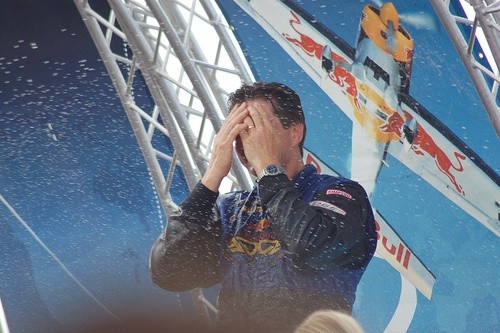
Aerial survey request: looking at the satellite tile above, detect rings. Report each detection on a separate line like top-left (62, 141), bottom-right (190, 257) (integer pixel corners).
top-left (246, 125), bottom-right (255, 131)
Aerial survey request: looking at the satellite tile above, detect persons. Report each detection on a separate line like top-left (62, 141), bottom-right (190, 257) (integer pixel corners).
top-left (149, 83), bottom-right (378, 333)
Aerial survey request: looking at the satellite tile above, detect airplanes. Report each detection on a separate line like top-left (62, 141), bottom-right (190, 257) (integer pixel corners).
top-left (232, 0), bottom-right (500, 302)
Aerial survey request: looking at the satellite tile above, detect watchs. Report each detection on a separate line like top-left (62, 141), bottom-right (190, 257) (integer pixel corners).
top-left (256, 163), bottom-right (288, 183)
